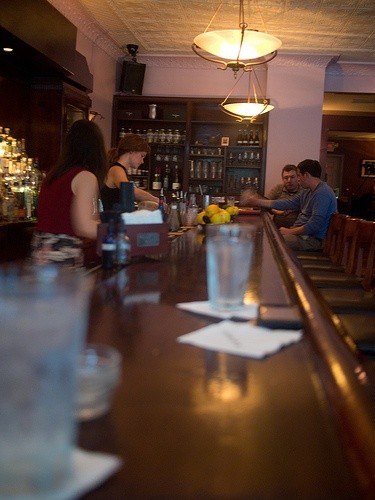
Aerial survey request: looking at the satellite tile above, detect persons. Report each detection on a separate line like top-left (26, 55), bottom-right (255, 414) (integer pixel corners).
top-left (240, 159), bottom-right (337, 250)
top-left (34, 119), bottom-right (106, 241)
top-left (264, 164), bottom-right (307, 229)
top-left (95, 134), bottom-right (165, 222)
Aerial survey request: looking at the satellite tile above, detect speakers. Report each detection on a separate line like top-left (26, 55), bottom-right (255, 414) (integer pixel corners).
top-left (119, 61), bottom-right (146, 95)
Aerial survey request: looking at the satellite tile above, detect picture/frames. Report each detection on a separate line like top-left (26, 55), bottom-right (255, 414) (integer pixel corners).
top-left (362, 160), bottom-right (375, 177)
top-left (221, 137), bottom-right (229, 146)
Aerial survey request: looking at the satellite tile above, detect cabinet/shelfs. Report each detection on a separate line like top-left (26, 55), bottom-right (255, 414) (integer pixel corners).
top-left (111, 95), bottom-right (270, 198)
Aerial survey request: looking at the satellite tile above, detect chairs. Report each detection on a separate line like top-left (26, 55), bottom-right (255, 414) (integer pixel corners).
top-left (291, 211), bottom-right (375, 352)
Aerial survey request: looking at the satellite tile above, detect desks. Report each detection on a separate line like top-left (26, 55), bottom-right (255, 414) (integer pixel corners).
top-left (0, 206), bottom-right (375, 500)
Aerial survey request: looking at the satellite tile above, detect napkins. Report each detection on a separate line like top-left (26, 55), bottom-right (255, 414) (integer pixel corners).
top-left (175, 319), bottom-right (302, 358)
top-left (175, 296), bottom-right (261, 323)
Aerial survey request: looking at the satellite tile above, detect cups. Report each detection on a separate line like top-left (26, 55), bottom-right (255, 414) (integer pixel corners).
top-left (0, 266), bottom-right (95, 497)
top-left (189, 193), bottom-right (196, 206)
top-left (204, 223), bottom-right (258, 312)
top-left (202, 195), bottom-right (209, 209)
top-left (226, 196), bottom-right (235, 207)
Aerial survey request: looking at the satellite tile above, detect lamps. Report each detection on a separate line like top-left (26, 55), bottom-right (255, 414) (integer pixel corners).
top-left (218, 65), bottom-right (276, 124)
top-left (192, 0), bottom-right (283, 77)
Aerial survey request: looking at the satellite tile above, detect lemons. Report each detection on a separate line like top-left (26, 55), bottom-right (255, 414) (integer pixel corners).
top-left (196, 204), bottom-right (238, 225)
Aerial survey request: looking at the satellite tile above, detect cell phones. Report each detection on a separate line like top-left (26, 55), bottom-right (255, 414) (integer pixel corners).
top-left (258, 303), bottom-right (303, 330)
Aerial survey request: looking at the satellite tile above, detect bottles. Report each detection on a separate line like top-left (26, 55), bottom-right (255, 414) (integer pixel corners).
top-left (95, 211), bottom-right (131, 269)
top-left (149, 104), bottom-right (157, 120)
top-left (0, 127), bottom-right (46, 221)
top-left (119, 127), bottom-right (261, 233)
